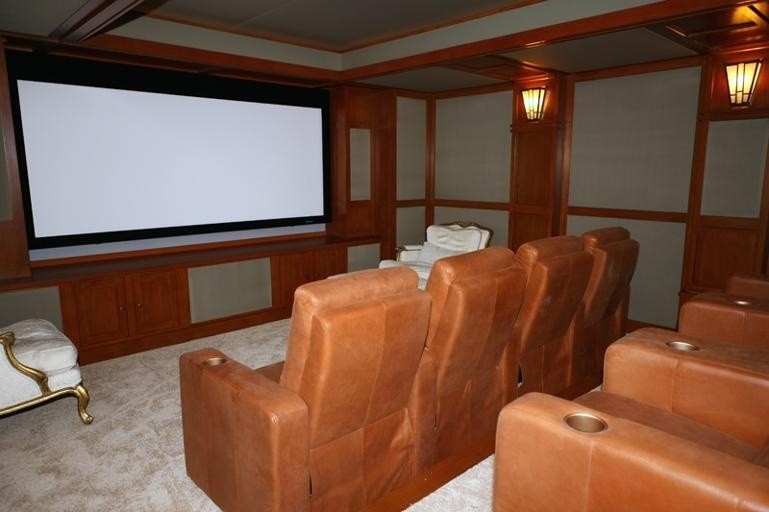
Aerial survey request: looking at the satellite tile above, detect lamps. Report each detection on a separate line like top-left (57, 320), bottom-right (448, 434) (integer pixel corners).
top-left (723, 59), bottom-right (765, 109)
top-left (521, 85), bottom-right (549, 123)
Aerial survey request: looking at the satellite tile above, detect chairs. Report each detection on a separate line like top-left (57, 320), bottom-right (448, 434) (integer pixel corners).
top-left (0, 316), bottom-right (94, 424)
top-left (411, 245), bottom-right (530, 506)
top-left (569, 225), bottom-right (641, 401)
top-left (377, 223), bottom-right (492, 291)
top-left (491, 335), bottom-right (768, 511)
top-left (690, 271), bottom-right (768, 311)
top-left (179, 267), bottom-right (433, 511)
top-left (516, 235), bottom-right (595, 400)
top-left (642, 297), bottom-right (768, 370)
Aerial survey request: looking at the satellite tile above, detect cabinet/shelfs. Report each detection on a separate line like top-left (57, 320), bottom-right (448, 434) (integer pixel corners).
top-left (59, 263), bottom-right (185, 353)
